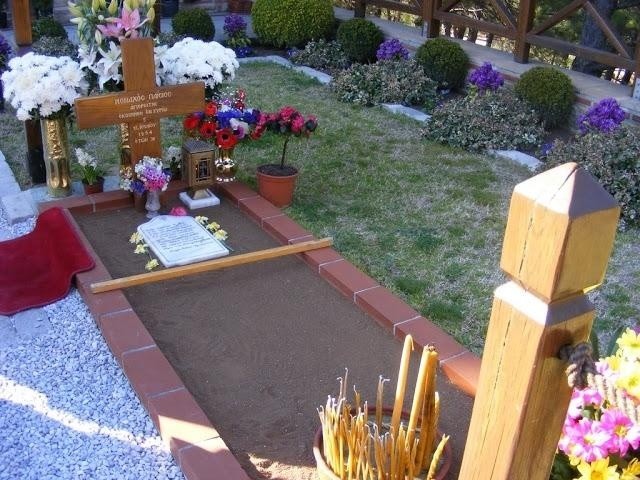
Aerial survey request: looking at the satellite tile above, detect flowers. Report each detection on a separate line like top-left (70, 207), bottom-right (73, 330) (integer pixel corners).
top-left (129, 231), bottom-right (159, 273)
top-left (169, 207), bottom-right (187, 216)
top-left (0, 0), bottom-right (318, 196)
top-left (195, 215), bottom-right (234, 253)
top-left (558, 327), bottom-right (640, 480)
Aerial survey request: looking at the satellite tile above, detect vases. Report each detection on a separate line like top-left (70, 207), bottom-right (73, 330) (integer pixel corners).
top-left (313, 406), bottom-right (453, 480)
top-left (257, 164), bottom-right (299, 210)
top-left (145, 192), bottom-right (161, 218)
top-left (134, 193), bottom-right (145, 211)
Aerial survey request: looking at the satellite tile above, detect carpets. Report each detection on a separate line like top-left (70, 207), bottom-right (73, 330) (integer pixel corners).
top-left (0, 207), bottom-right (95, 315)
